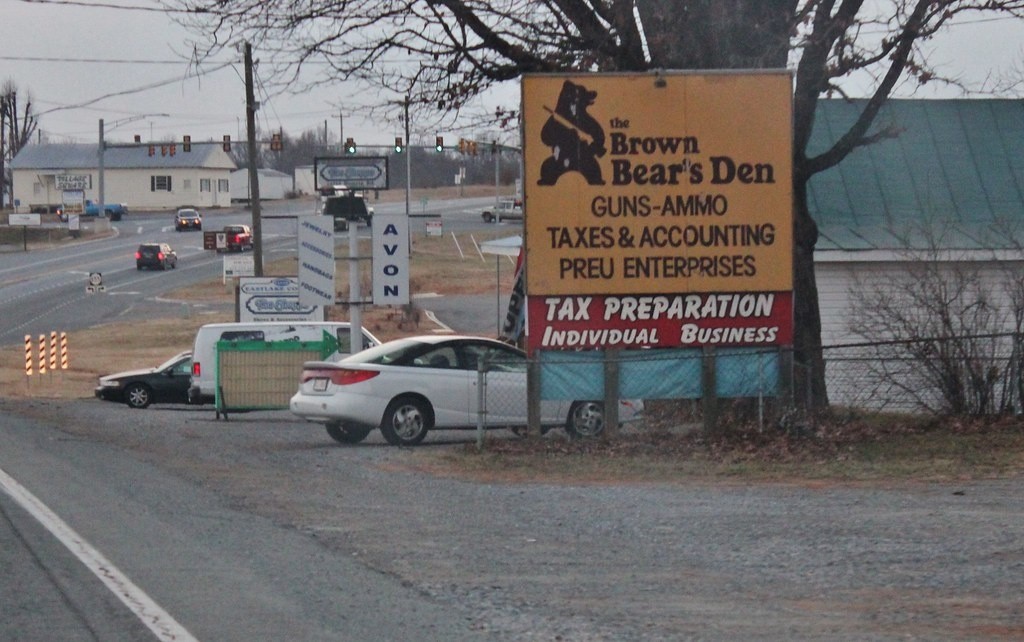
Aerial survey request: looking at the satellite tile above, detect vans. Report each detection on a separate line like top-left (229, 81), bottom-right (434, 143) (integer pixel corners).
top-left (188, 320), bottom-right (424, 405)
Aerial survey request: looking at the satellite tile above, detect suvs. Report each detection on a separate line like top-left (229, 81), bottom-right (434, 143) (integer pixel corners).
top-left (137, 243), bottom-right (177, 271)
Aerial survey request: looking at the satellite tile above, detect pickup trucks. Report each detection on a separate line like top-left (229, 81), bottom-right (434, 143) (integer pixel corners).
top-left (56, 198), bottom-right (128, 221)
top-left (217, 224), bottom-right (253, 254)
top-left (479, 200), bottom-right (522, 223)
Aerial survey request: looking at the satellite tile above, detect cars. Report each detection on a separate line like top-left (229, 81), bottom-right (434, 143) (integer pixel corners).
top-left (95, 350), bottom-right (194, 410)
top-left (174, 209), bottom-right (202, 232)
top-left (288, 334), bottom-right (646, 447)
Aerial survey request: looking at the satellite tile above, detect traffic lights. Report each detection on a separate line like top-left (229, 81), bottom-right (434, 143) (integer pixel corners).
top-left (345, 138), bottom-right (356, 153)
top-left (436, 136), bottom-right (443, 152)
top-left (395, 137), bottom-right (402, 153)
